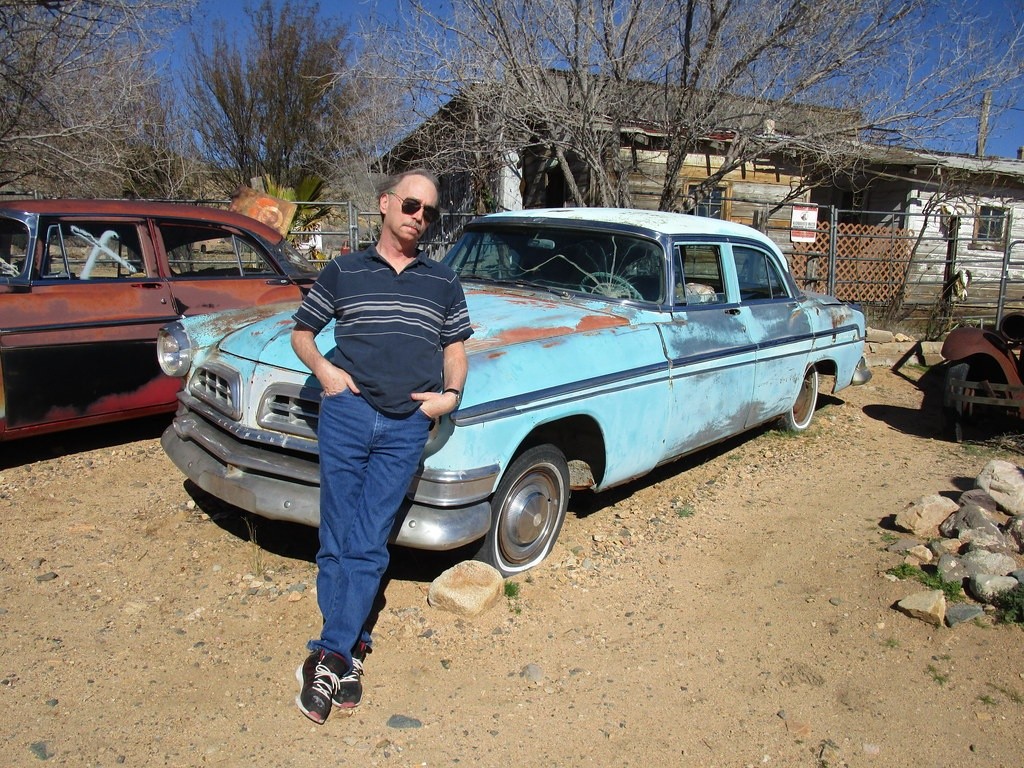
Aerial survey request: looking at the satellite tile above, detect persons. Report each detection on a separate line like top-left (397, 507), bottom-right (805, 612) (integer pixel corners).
top-left (290, 168), bottom-right (476, 725)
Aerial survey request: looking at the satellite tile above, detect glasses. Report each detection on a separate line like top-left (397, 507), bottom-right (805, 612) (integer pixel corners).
top-left (389, 191), bottom-right (441, 222)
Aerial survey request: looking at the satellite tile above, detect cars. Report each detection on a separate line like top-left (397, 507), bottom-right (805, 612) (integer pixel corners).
top-left (0, 191), bottom-right (318, 443)
top-left (157, 207), bottom-right (873, 579)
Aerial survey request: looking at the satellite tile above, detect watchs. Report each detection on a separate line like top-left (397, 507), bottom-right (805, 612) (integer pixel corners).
top-left (441, 388), bottom-right (461, 407)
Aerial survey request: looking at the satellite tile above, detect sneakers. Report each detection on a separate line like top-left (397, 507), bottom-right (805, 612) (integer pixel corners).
top-left (332, 639), bottom-right (372, 708)
top-left (294, 648), bottom-right (350, 725)
